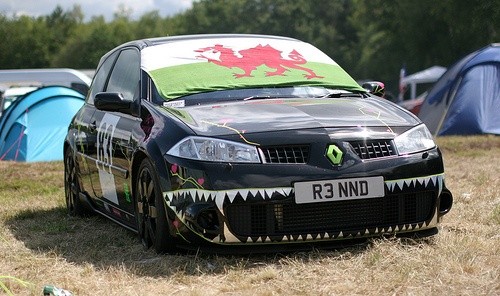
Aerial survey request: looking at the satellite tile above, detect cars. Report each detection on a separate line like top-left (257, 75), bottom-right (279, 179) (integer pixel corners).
top-left (61, 32), bottom-right (453, 256)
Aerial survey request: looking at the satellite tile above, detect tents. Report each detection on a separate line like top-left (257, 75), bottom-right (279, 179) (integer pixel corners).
top-left (0, 85), bottom-right (88, 163)
top-left (401, 65), bottom-right (449, 100)
top-left (418, 42), bottom-right (500, 137)
